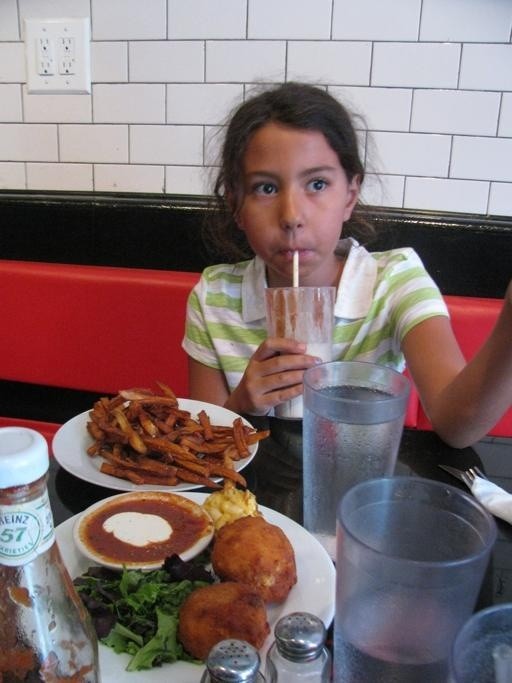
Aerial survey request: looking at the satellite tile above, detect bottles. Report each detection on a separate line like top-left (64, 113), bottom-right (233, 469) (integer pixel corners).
top-left (0, 426), bottom-right (98, 683)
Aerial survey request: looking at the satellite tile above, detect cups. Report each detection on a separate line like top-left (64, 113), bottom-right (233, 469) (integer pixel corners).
top-left (264, 286), bottom-right (335, 425)
top-left (449, 605), bottom-right (511, 683)
top-left (294, 361), bottom-right (412, 566)
top-left (331, 476), bottom-right (499, 681)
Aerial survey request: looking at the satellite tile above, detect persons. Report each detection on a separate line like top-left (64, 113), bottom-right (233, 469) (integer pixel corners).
top-left (179, 76), bottom-right (512, 453)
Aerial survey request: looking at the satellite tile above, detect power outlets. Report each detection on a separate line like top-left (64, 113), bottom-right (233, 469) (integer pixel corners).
top-left (23, 17), bottom-right (92, 96)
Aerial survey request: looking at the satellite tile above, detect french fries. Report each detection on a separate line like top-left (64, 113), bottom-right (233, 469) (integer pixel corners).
top-left (87, 376), bottom-right (272, 490)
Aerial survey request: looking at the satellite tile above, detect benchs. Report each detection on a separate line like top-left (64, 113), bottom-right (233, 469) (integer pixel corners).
top-left (1, 188), bottom-right (512, 452)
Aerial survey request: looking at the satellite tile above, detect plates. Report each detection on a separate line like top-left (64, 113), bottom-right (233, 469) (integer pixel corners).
top-left (51, 398), bottom-right (261, 492)
top-left (52, 488), bottom-right (335, 680)
